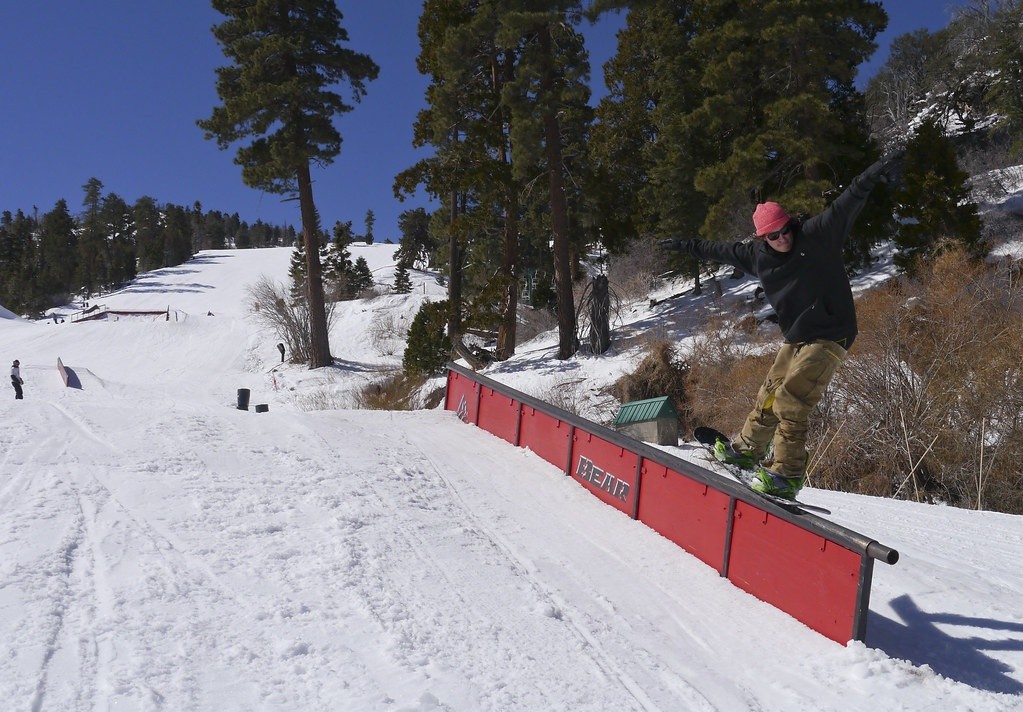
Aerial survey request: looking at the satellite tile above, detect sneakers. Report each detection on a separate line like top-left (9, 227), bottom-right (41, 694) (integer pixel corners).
top-left (714, 438), bottom-right (769, 470)
top-left (751, 451), bottom-right (809, 501)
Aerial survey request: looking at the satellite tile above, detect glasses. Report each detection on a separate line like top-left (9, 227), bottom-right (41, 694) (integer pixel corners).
top-left (764, 223), bottom-right (789, 241)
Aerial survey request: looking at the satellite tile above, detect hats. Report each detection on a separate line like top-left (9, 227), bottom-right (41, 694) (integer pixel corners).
top-left (752, 201), bottom-right (790, 236)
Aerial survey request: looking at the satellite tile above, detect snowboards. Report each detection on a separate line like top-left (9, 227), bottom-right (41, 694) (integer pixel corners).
top-left (692, 425), bottom-right (834, 516)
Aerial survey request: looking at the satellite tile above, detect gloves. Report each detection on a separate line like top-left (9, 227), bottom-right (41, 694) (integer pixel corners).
top-left (857, 150), bottom-right (903, 191)
top-left (655, 238), bottom-right (688, 253)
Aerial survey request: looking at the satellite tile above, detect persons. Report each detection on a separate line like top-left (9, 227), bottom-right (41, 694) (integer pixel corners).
top-left (61, 318), bottom-right (65, 323)
top-left (53, 313), bottom-right (58, 324)
top-left (10, 360), bottom-right (23, 399)
top-left (656, 150), bottom-right (905, 499)
